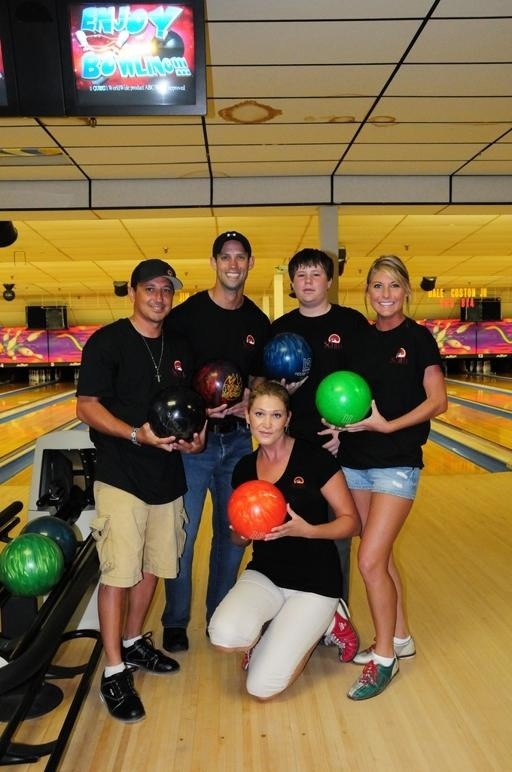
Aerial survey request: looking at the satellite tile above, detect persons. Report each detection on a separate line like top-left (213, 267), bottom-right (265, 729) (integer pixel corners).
top-left (336, 254), bottom-right (449, 700)
top-left (161, 229), bottom-right (274, 654)
top-left (272, 249), bottom-right (372, 643)
top-left (74, 257), bottom-right (210, 725)
top-left (207, 380), bottom-right (360, 701)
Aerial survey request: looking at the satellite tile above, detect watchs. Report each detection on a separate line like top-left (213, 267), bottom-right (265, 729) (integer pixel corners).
top-left (130, 428), bottom-right (142, 447)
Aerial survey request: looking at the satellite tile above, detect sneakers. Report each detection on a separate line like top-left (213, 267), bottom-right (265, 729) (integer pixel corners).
top-left (348, 658), bottom-right (401, 699)
top-left (355, 638), bottom-right (418, 663)
top-left (98, 668), bottom-right (146, 723)
top-left (123, 637), bottom-right (181, 675)
top-left (325, 600), bottom-right (357, 663)
top-left (163, 627), bottom-right (191, 655)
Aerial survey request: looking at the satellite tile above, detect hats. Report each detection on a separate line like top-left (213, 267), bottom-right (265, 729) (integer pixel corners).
top-left (214, 232), bottom-right (252, 254)
top-left (132, 260), bottom-right (185, 291)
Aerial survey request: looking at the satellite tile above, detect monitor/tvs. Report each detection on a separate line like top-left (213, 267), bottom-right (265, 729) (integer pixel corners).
top-left (57, 1), bottom-right (207, 117)
top-left (1, 7), bottom-right (20, 120)
top-left (26, 306), bottom-right (67, 331)
top-left (461, 299), bottom-right (502, 322)
